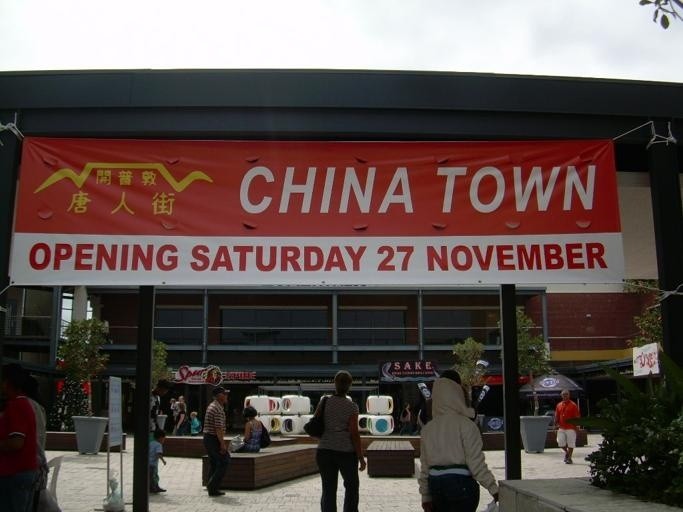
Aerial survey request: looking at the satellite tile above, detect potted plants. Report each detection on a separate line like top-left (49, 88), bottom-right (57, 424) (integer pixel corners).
top-left (149, 337), bottom-right (168, 432)
top-left (515, 306), bottom-right (558, 454)
top-left (56, 317), bottom-right (112, 455)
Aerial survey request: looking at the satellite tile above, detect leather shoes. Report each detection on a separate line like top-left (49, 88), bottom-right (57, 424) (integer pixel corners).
top-left (207, 482), bottom-right (225, 496)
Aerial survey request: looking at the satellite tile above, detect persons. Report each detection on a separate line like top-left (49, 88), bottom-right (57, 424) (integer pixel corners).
top-left (174, 394), bottom-right (186, 419)
top-left (553, 390), bottom-right (579, 464)
top-left (313, 370), bottom-right (367, 511)
top-left (0, 362), bottom-right (39, 512)
top-left (399, 403), bottom-right (413, 436)
top-left (23, 375), bottom-right (49, 512)
top-left (203, 387), bottom-right (231, 495)
top-left (147, 429), bottom-right (167, 492)
top-left (419, 371), bottom-right (476, 425)
top-left (413, 404), bottom-right (424, 436)
top-left (419, 379), bottom-right (499, 510)
top-left (147, 379), bottom-right (170, 492)
top-left (230, 407), bottom-right (262, 452)
top-left (172, 411), bottom-right (190, 435)
top-left (188, 411), bottom-right (201, 435)
top-left (169, 397), bottom-right (178, 416)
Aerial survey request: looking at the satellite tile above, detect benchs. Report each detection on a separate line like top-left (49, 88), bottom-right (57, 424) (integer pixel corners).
top-left (163, 433), bottom-right (321, 491)
top-left (480, 429), bottom-right (586, 451)
top-left (360, 434), bottom-right (421, 479)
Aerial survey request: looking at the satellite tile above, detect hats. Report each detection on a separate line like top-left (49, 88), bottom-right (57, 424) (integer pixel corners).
top-left (212, 387), bottom-right (230, 396)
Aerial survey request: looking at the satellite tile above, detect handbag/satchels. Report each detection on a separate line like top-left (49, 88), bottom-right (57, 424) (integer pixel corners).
top-left (195, 425), bottom-right (203, 432)
top-left (303, 396), bottom-right (327, 438)
top-left (260, 422), bottom-right (272, 448)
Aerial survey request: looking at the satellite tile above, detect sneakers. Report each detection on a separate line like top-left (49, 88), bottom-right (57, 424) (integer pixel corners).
top-left (564, 453), bottom-right (572, 464)
top-left (150, 484), bottom-right (167, 492)
